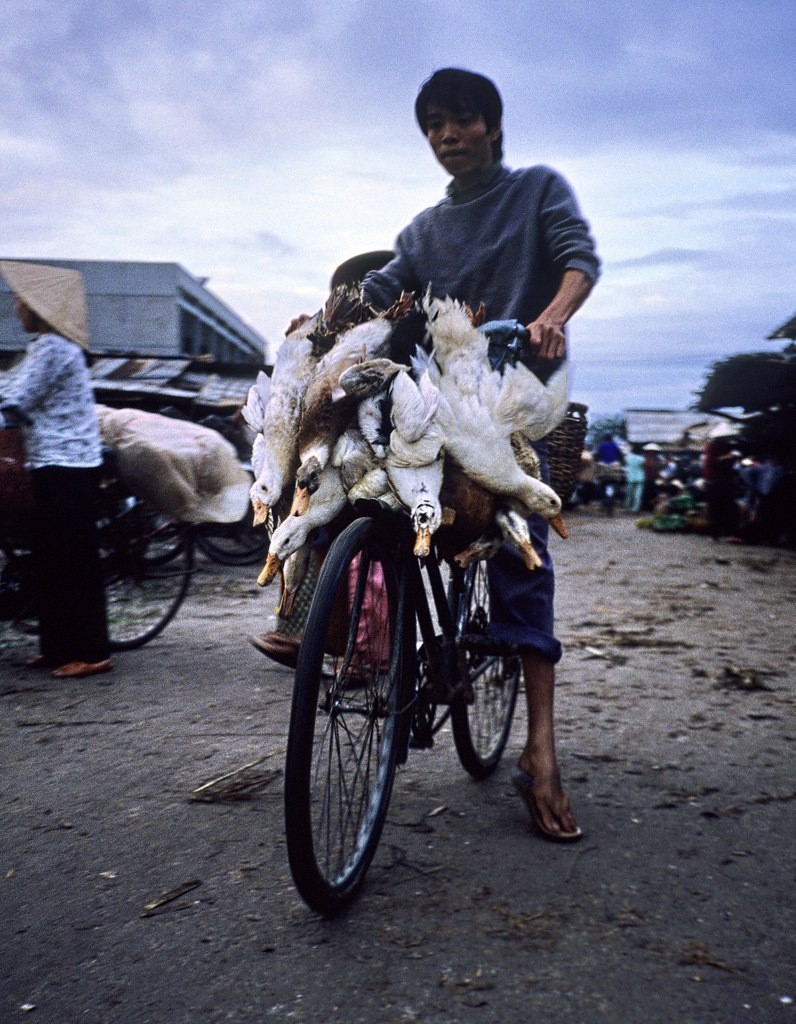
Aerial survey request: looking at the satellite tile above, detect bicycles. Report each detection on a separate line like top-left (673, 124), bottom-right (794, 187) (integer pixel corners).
top-left (280, 316), bottom-right (537, 920)
top-left (1, 443), bottom-right (271, 652)
top-left (592, 461), bottom-right (629, 516)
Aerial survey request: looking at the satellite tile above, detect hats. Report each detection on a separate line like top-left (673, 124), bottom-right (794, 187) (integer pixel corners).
top-left (643, 443), bottom-right (662, 452)
top-left (709, 423), bottom-right (740, 438)
top-left (0, 260), bottom-right (89, 352)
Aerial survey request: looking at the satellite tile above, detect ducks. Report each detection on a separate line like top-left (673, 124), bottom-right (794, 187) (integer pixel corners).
top-left (241, 280), bottom-right (573, 619)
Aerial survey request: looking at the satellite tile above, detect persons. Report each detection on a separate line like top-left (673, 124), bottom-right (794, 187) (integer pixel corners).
top-left (643, 443), bottom-right (662, 511)
top-left (702, 423), bottom-right (740, 544)
top-left (246, 69), bottom-right (603, 840)
top-left (0, 262), bottom-right (116, 677)
top-left (624, 447), bottom-right (645, 512)
top-left (594, 430), bottom-right (622, 462)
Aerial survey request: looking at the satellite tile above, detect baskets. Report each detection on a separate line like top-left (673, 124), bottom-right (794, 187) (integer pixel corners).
top-left (541, 403), bottom-right (588, 505)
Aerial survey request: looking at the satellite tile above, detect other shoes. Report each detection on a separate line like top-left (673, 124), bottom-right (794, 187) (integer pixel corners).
top-left (51, 657), bottom-right (112, 676)
top-left (25, 654), bottom-right (69, 665)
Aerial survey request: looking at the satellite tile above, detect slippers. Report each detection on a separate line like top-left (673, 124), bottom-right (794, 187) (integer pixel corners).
top-left (246, 632), bottom-right (302, 668)
top-left (512, 765), bottom-right (583, 841)
